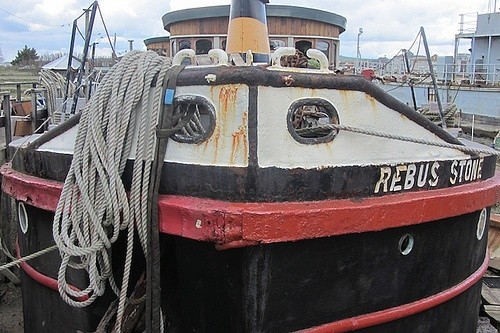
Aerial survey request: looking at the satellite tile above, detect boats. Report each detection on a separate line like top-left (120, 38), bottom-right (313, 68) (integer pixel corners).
top-left (0, 0), bottom-right (499, 333)
